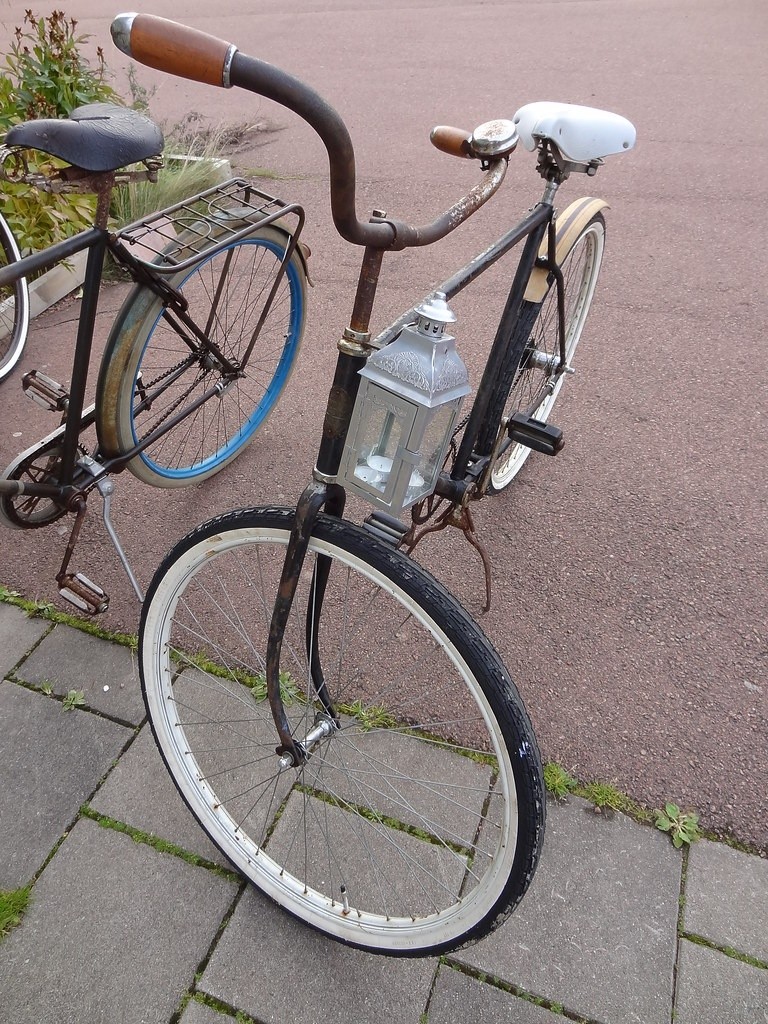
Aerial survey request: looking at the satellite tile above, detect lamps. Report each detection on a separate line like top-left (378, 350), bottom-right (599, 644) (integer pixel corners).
top-left (331, 290), bottom-right (474, 519)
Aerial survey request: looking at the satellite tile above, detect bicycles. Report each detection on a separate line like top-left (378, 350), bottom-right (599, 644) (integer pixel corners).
top-left (108, 10), bottom-right (638, 958)
top-left (0, 102), bottom-right (316, 616)
